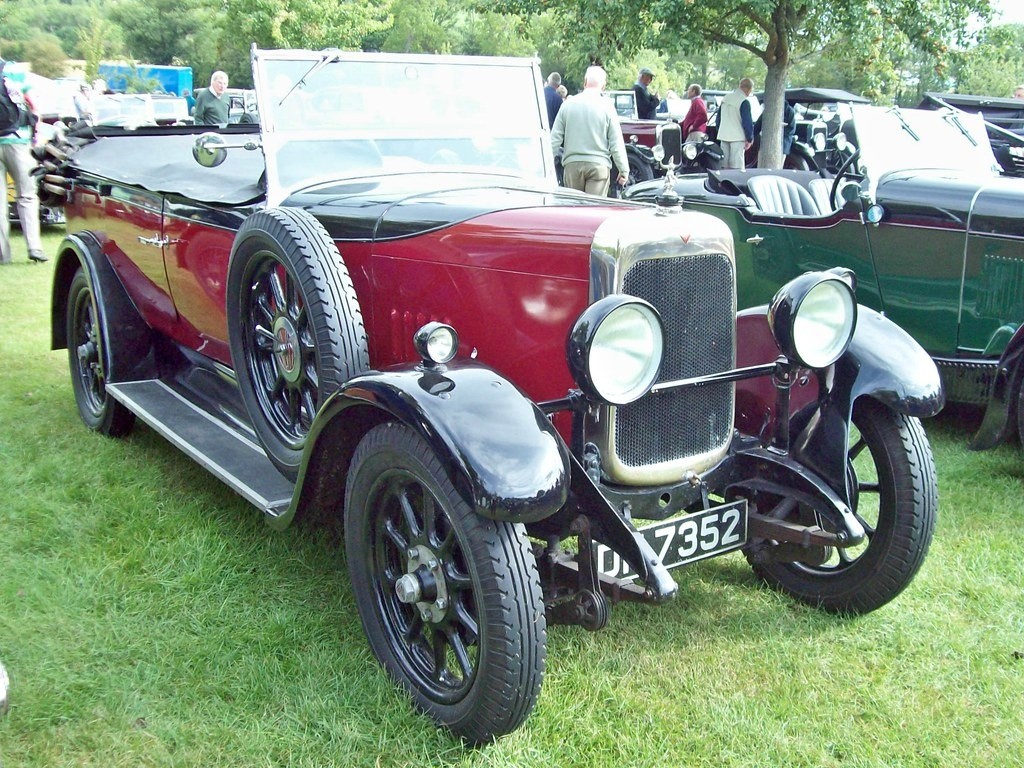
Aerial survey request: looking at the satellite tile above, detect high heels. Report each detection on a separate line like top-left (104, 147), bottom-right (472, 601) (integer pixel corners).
top-left (28, 249), bottom-right (48, 262)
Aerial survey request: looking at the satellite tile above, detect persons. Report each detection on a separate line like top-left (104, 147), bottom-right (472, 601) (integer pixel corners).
top-left (624, 67), bottom-right (664, 118)
top-left (715, 77), bottom-right (755, 169)
top-left (194, 70), bottom-right (233, 129)
top-left (753, 96), bottom-right (797, 170)
top-left (548, 65), bottom-right (630, 198)
top-left (1, 56), bottom-right (49, 265)
top-left (545, 57), bottom-right (685, 119)
top-left (19, 76), bottom-right (197, 125)
top-left (679, 83), bottom-right (709, 140)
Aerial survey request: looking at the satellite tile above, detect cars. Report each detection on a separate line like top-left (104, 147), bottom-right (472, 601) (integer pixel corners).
top-left (1, 57), bottom-right (743, 226)
top-left (617, 100), bottom-right (1024, 452)
top-left (918, 87), bottom-right (1024, 180)
top-left (32, 46), bottom-right (947, 739)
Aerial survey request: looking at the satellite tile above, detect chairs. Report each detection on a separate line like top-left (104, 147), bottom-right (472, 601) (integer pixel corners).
top-left (808, 178), bottom-right (847, 216)
top-left (747, 175), bottom-right (821, 216)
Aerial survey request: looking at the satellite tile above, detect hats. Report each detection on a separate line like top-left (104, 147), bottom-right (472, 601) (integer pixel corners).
top-left (640, 68), bottom-right (655, 77)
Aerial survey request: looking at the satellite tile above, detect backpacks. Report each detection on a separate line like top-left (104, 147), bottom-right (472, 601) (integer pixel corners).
top-left (0, 75), bottom-right (38, 139)
